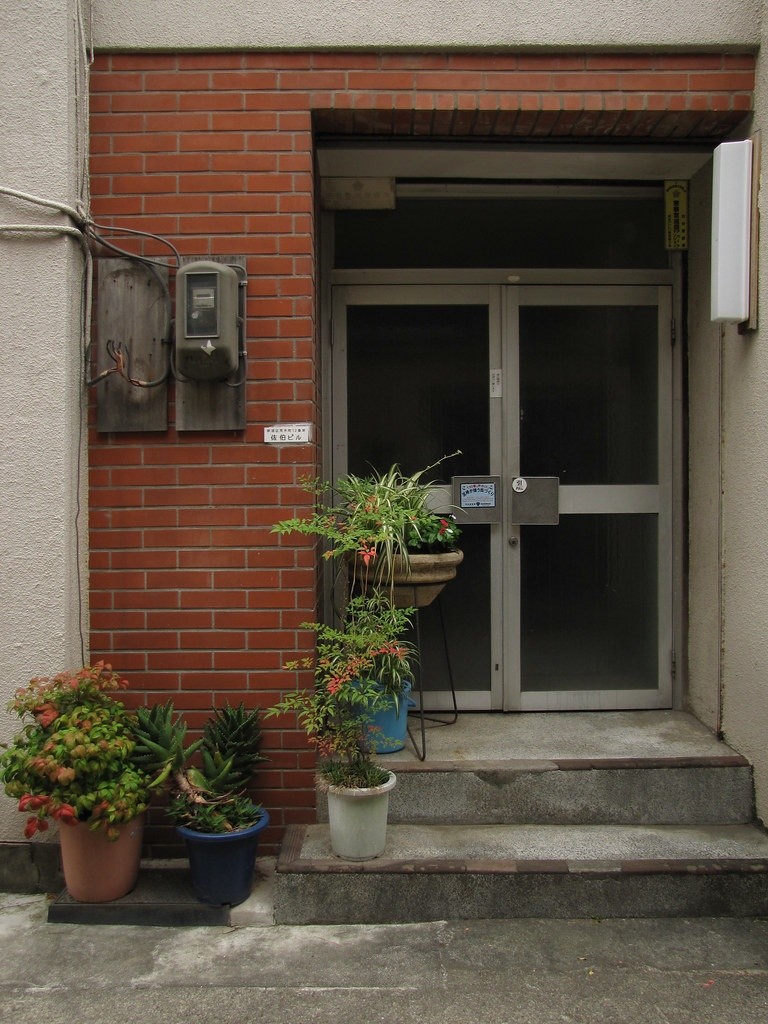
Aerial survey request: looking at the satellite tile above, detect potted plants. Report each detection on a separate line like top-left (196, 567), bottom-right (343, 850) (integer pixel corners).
top-left (0, 660), bottom-right (154, 904)
top-left (265, 641), bottom-right (410, 862)
top-left (121, 695), bottom-right (270, 908)
top-left (269, 449), bottom-right (469, 609)
top-left (300, 595), bottom-right (421, 754)
